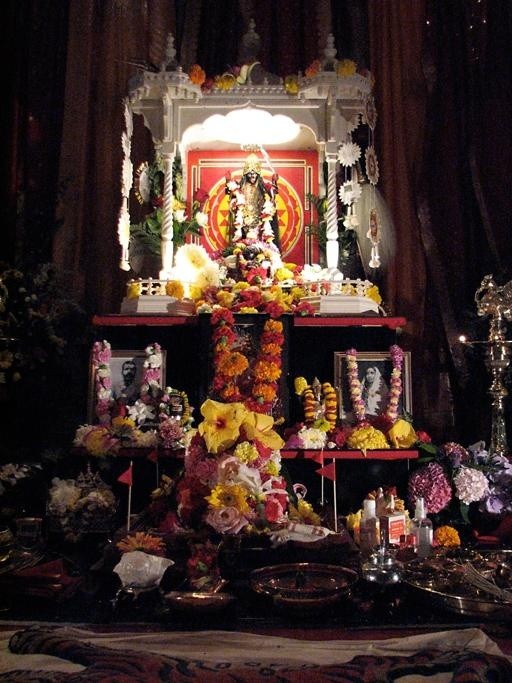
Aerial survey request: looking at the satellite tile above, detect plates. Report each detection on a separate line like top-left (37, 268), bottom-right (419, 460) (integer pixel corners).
top-left (249, 562), bottom-right (358, 601)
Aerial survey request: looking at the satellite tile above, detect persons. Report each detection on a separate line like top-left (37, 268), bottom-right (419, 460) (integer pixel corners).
top-left (110, 360), bottom-right (140, 401)
top-left (231, 153), bottom-right (278, 244)
top-left (360, 366), bottom-right (387, 417)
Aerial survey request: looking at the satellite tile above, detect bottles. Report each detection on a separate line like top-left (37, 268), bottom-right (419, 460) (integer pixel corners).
top-left (357, 500), bottom-right (380, 569)
top-left (411, 497), bottom-right (434, 552)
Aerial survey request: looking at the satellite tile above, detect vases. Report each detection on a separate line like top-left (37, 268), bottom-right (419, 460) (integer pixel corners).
top-left (14, 363), bottom-right (62, 422)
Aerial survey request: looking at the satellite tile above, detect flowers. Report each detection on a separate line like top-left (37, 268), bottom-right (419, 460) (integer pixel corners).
top-left (1, 464), bottom-right (32, 489)
top-left (117, 401), bottom-right (338, 553)
top-left (48, 471), bottom-right (117, 533)
top-left (409, 431), bottom-right (511, 548)
top-left (72, 341), bottom-right (192, 456)
top-left (131, 158), bottom-right (209, 255)
top-left (211, 290), bottom-right (285, 415)
top-left (165, 242), bottom-right (380, 309)
top-left (1, 265), bottom-right (83, 382)
top-left (282, 346), bottom-right (419, 451)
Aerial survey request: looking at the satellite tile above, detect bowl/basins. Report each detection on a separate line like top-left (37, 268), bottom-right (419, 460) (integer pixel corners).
top-left (400, 549), bottom-right (511, 616)
